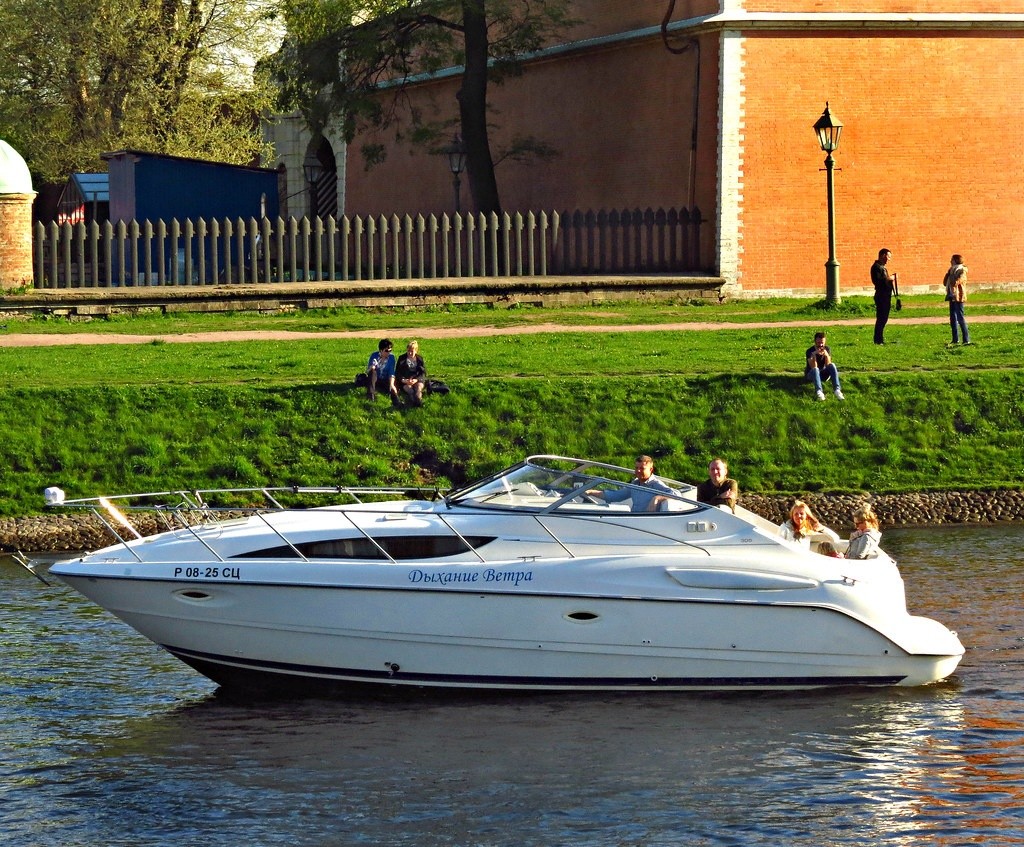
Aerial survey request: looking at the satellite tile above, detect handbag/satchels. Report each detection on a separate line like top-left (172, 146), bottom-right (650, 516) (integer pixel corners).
top-left (356, 373), bottom-right (368, 386)
top-left (427, 379), bottom-right (449, 396)
top-left (896, 300), bottom-right (901, 310)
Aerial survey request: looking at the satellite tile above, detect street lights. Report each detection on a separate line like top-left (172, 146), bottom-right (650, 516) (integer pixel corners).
top-left (447, 131), bottom-right (468, 215)
top-left (302, 147), bottom-right (325, 272)
top-left (814, 101), bottom-right (845, 305)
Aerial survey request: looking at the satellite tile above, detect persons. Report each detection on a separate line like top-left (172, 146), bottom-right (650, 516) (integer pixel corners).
top-left (871, 249), bottom-right (897, 345)
top-left (844, 507), bottom-right (882, 560)
top-left (777, 501), bottom-right (840, 551)
top-left (697, 458), bottom-right (738, 506)
top-left (943, 254), bottom-right (970, 346)
top-left (817, 542), bottom-right (844, 559)
top-left (584, 455), bottom-right (681, 512)
top-left (366, 338), bottom-right (398, 402)
top-left (395, 341), bottom-right (426, 408)
top-left (804, 333), bottom-right (845, 402)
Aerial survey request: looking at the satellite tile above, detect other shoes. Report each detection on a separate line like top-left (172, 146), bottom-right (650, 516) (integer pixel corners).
top-left (392, 394), bottom-right (401, 406)
top-left (815, 390), bottom-right (825, 401)
top-left (367, 394), bottom-right (374, 400)
top-left (407, 393), bottom-right (421, 406)
top-left (834, 390), bottom-right (845, 399)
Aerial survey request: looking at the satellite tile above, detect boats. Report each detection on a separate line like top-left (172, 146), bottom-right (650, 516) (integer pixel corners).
top-left (12, 455), bottom-right (967, 699)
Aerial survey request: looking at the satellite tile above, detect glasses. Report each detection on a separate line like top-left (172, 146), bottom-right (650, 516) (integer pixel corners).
top-left (855, 522), bottom-right (864, 527)
top-left (385, 349), bottom-right (392, 352)
